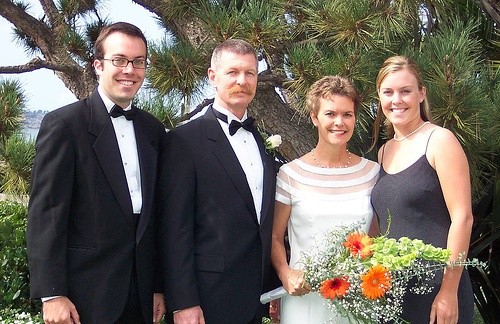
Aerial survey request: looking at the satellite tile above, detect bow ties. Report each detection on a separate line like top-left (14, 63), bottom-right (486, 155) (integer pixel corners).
top-left (211, 107), bottom-right (255, 137)
top-left (108, 104), bottom-right (136, 121)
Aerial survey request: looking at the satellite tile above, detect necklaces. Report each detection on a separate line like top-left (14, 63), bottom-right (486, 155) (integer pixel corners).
top-left (311, 149), bottom-right (351, 168)
top-left (394, 121), bottom-right (430, 141)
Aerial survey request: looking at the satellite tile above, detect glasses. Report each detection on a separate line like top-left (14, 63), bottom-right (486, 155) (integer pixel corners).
top-left (99, 54), bottom-right (147, 69)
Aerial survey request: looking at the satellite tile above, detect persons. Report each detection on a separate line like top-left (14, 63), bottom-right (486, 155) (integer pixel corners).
top-left (26, 23), bottom-right (166, 324)
top-left (270, 75), bottom-right (380, 324)
top-left (157, 38), bottom-right (283, 324)
top-left (370, 56), bottom-right (474, 324)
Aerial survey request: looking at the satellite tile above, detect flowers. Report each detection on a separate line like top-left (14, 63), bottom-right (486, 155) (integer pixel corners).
top-left (261, 204), bottom-right (490, 324)
top-left (263, 134), bottom-right (282, 156)
